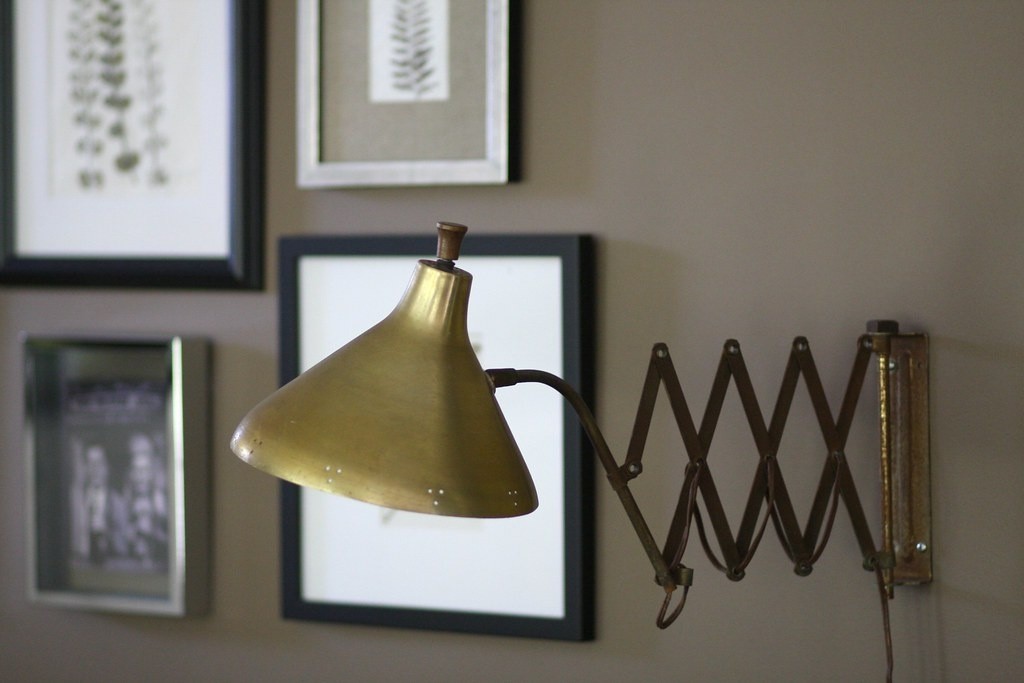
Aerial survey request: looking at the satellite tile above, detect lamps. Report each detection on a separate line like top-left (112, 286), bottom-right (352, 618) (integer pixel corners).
top-left (230, 218), bottom-right (936, 627)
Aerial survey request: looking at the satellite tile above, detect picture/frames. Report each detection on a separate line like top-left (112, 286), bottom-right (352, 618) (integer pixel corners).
top-left (293, 0), bottom-right (520, 189)
top-left (20, 334), bottom-right (210, 619)
top-left (0, 0), bottom-right (268, 295)
top-left (275, 232), bottom-right (597, 645)
